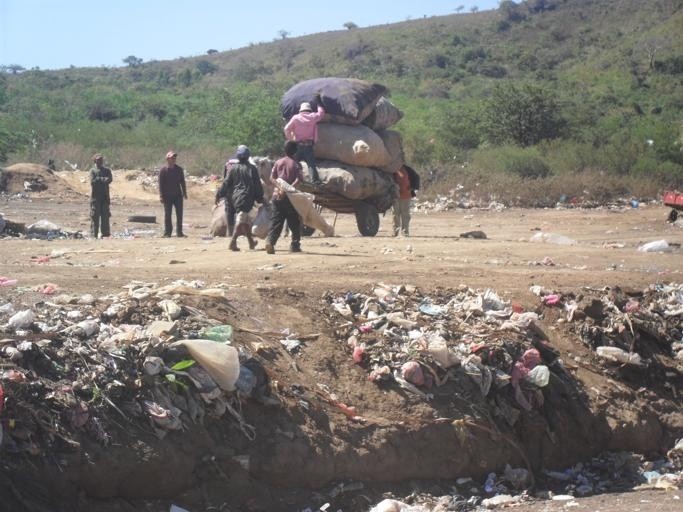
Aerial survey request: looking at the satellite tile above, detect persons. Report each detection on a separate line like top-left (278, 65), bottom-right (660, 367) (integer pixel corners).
top-left (158, 150), bottom-right (188, 238)
top-left (282, 101), bottom-right (326, 188)
top-left (213, 144), bottom-right (266, 252)
top-left (87, 153), bottom-right (113, 240)
top-left (263, 140), bottom-right (304, 255)
top-left (387, 164), bottom-right (411, 238)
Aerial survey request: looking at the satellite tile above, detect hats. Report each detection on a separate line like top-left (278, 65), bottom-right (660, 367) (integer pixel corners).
top-left (237, 144), bottom-right (250, 159)
top-left (166, 151), bottom-right (177, 159)
top-left (299, 103), bottom-right (312, 111)
top-left (92, 153), bottom-right (104, 160)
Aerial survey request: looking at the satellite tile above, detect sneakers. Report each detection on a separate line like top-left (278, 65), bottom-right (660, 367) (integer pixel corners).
top-left (228, 241), bottom-right (303, 253)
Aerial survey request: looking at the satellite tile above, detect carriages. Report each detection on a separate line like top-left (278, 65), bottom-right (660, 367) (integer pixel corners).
top-left (251, 157), bottom-right (381, 237)
